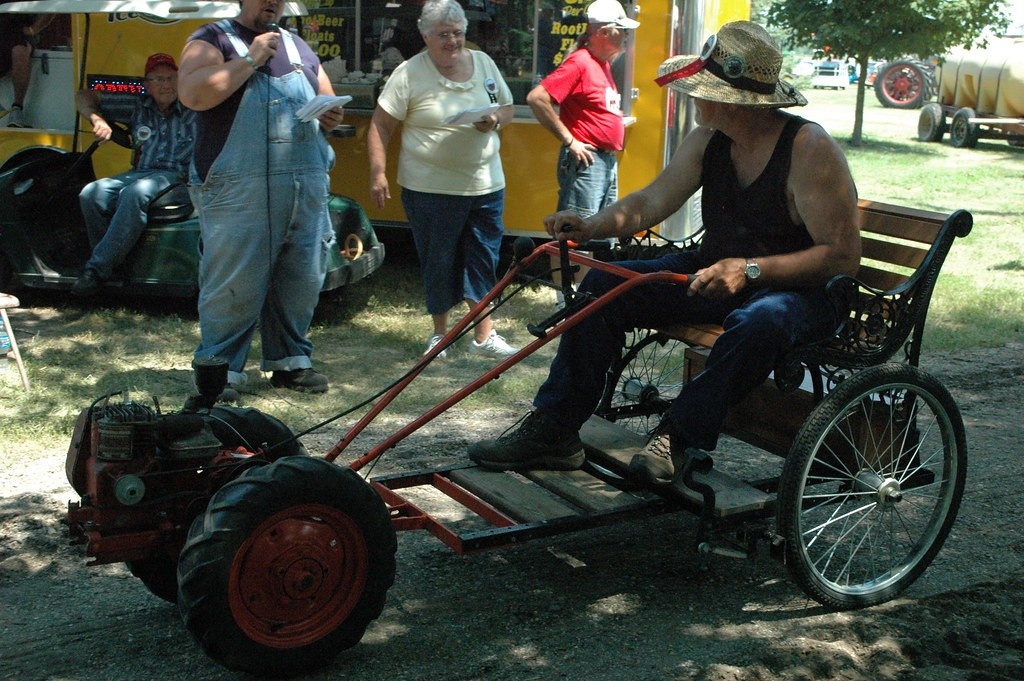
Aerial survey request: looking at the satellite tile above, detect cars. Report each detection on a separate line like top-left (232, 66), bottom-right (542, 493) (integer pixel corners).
top-left (791, 56), bottom-right (887, 91)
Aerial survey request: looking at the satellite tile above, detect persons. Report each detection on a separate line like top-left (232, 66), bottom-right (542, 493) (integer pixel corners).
top-left (177, 0), bottom-right (344, 410)
top-left (527, 1), bottom-right (640, 317)
top-left (367, 0), bottom-right (520, 360)
top-left (73, 53), bottom-right (197, 296)
top-left (468, 18), bottom-right (862, 483)
top-left (0, 13), bottom-right (57, 127)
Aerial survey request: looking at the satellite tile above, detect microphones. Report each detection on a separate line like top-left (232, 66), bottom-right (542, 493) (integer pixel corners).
top-left (264, 22), bottom-right (279, 68)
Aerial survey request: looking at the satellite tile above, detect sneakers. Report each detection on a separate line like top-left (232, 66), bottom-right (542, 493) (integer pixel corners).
top-left (467, 410), bottom-right (586, 472)
top-left (215, 382), bottom-right (241, 402)
top-left (270, 368), bottom-right (329, 392)
top-left (423, 334), bottom-right (456, 359)
top-left (6, 103), bottom-right (24, 129)
top-left (629, 413), bottom-right (700, 486)
top-left (469, 329), bottom-right (521, 359)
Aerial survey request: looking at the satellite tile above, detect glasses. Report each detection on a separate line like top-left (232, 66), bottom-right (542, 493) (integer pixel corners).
top-left (599, 24), bottom-right (631, 34)
top-left (145, 75), bottom-right (178, 86)
top-left (428, 28), bottom-right (467, 40)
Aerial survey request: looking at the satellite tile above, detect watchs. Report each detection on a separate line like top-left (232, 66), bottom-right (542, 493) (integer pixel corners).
top-left (744, 257), bottom-right (762, 287)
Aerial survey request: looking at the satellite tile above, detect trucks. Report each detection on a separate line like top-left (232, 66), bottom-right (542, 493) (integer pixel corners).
top-left (0, 0), bottom-right (756, 252)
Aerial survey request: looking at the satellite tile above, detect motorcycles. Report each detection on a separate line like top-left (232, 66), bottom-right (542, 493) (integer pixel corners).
top-left (0, 134), bottom-right (389, 306)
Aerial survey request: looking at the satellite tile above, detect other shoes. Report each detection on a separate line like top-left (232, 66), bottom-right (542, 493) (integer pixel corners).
top-left (71, 268), bottom-right (104, 297)
top-left (554, 301), bottom-right (566, 324)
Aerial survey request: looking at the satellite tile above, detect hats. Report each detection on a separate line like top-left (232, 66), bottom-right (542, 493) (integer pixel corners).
top-left (654, 25), bottom-right (806, 108)
top-left (145, 53), bottom-right (178, 75)
top-left (587, 0), bottom-right (640, 29)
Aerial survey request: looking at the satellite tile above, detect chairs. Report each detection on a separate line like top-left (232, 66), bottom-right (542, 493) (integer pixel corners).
top-left (601, 196), bottom-right (974, 481)
top-left (0, 294), bottom-right (29, 392)
